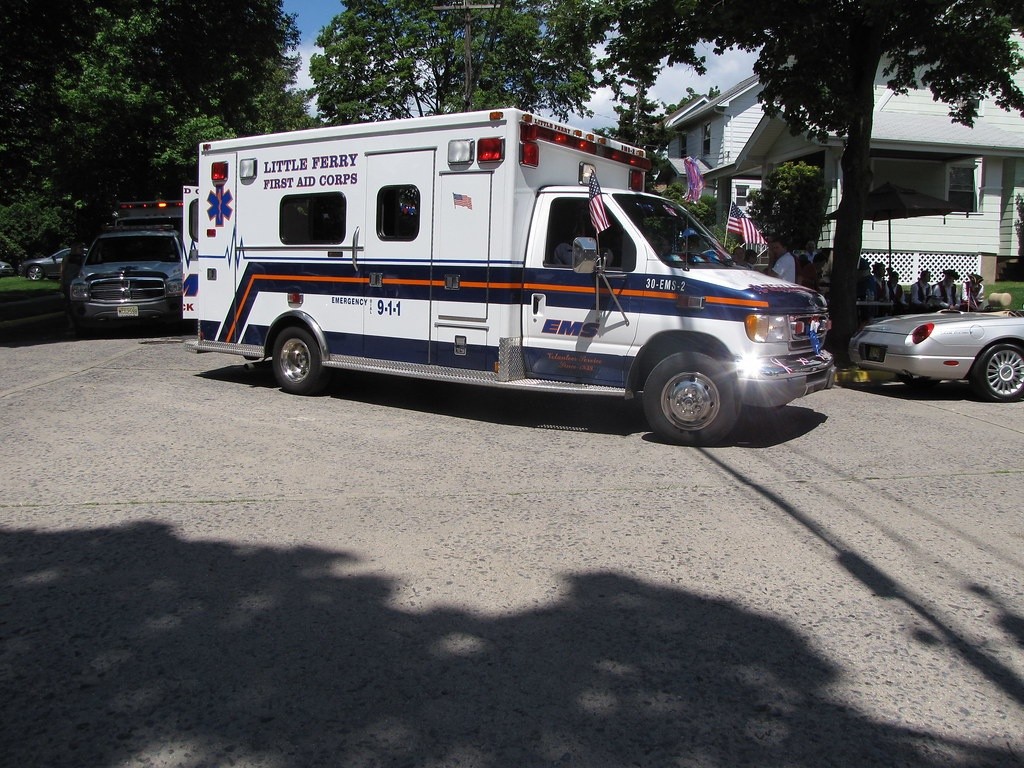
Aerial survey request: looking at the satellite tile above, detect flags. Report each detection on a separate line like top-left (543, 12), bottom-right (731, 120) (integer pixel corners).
top-left (452, 192), bottom-right (473, 210)
top-left (727, 201), bottom-right (768, 246)
top-left (588, 170), bottom-right (611, 233)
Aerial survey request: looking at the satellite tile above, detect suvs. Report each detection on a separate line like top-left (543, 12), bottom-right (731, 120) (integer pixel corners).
top-left (68, 223), bottom-right (186, 336)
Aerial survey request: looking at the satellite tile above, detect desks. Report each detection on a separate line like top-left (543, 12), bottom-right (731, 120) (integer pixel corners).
top-left (856, 301), bottom-right (894, 321)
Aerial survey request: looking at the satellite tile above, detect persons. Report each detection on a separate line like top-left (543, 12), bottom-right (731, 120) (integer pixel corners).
top-left (59, 242), bottom-right (86, 316)
top-left (555, 220), bottom-right (612, 269)
top-left (731, 242), bottom-right (985, 320)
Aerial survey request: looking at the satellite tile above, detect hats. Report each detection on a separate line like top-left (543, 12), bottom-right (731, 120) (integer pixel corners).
top-left (943, 269), bottom-right (959, 280)
top-left (746, 249), bottom-right (758, 256)
top-left (968, 275), bottom-right (984, 284)
top-left (682, 228), bottom-right (701, 240)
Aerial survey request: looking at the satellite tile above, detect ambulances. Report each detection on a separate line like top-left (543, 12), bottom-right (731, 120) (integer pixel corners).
top-left (179, 106), bottom-right (838, 448)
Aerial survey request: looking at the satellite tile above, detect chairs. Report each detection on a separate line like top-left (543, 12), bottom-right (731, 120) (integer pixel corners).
top-left (989, 292), bottom-right (1012, 311)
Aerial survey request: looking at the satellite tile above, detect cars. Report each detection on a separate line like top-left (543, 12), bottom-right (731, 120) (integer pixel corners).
top-left (0, 260), bottom-right (15, 277)
top-left (17, 246), bottom-right (102, 281)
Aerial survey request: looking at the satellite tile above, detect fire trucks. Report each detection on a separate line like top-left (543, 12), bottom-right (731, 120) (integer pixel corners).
top-left (113, 199), bottom-right (184, 232)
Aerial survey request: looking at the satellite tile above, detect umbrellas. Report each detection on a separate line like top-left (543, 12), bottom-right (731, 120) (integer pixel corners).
top-left (826, 182), bottom-right (956, 266)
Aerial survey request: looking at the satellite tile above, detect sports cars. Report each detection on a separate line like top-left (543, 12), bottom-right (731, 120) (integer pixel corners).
top-left (848, 293), bottom-right (1024, 403)
